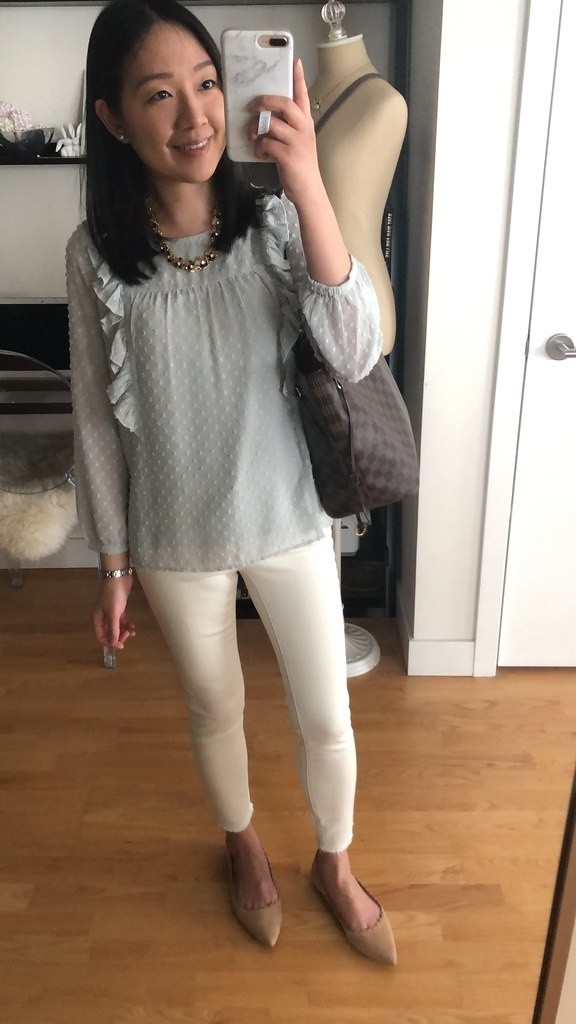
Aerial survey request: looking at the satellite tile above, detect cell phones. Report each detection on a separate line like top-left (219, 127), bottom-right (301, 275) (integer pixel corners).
top-left (221, 28), bottom-right (293, 163)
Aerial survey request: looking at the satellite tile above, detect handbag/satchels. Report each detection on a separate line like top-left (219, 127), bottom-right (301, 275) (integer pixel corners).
top-left (294, 320), bottom-right (417, 518)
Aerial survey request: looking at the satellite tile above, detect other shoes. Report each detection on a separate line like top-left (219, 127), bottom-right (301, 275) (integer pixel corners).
top-left (226, 840), bottom-right (284, 951)
top-left (310, 853), bottom-right (398, 966)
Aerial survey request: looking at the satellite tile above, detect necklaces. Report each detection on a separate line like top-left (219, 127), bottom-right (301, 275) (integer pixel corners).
top-left (146, 197), bottom-right (221, 273)
top-left (312, 62), bottom-right (372, 109)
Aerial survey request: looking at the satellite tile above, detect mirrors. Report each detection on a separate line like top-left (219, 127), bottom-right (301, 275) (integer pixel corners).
top-left (0, 2), bottom-right (576, 1023)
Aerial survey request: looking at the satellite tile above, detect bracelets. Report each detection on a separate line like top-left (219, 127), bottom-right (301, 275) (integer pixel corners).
top-left (103, 568), bottom-right (133, 578)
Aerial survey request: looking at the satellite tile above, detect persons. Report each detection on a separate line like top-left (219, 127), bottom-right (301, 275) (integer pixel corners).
top-left (297, 34), bottom-right (409, 356)
top-left (62, 0), bottom-right (398, 966)
